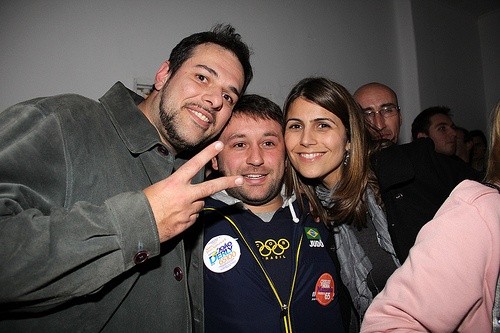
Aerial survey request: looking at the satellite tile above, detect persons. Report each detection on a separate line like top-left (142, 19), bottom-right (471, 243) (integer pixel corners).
top-left (353, 82), bottom-right (446, 265)
top-left (284, 76), bottom-right (446, 333)
top-left (204, 93), bottom-right (361, 333)
top-left (411, 106), bottom-right (476, 200)
top-left (0, 22), bottom-right (253, 333)
top-left (469, 130), bottom-right (488, 183)
top-left (455, 126), bottom-right (474, 164)
top-left (360, 94), bottom-right (500, 333)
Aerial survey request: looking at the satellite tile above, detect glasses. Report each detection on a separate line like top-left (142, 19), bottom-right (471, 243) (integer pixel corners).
top-left (364, 105), bottom-right (400, 118)
